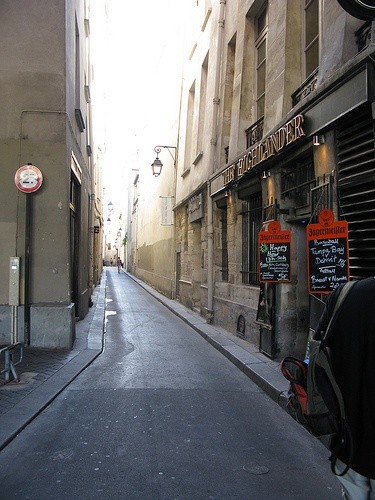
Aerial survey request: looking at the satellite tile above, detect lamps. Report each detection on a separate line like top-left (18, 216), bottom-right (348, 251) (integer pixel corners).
top-left (151, 145), bottom-right (177, 178)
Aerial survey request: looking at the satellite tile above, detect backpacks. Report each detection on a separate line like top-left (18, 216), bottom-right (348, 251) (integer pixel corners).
top-left (279, 278), bottom-right (356, 478)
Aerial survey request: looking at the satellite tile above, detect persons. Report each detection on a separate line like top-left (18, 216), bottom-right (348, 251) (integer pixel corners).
top-left (326, 449), bottom-right (374, 500)
top-left (109, 258), bottom-right (113, 267)
top-left (116, 257), bottom-right (121, 273)
